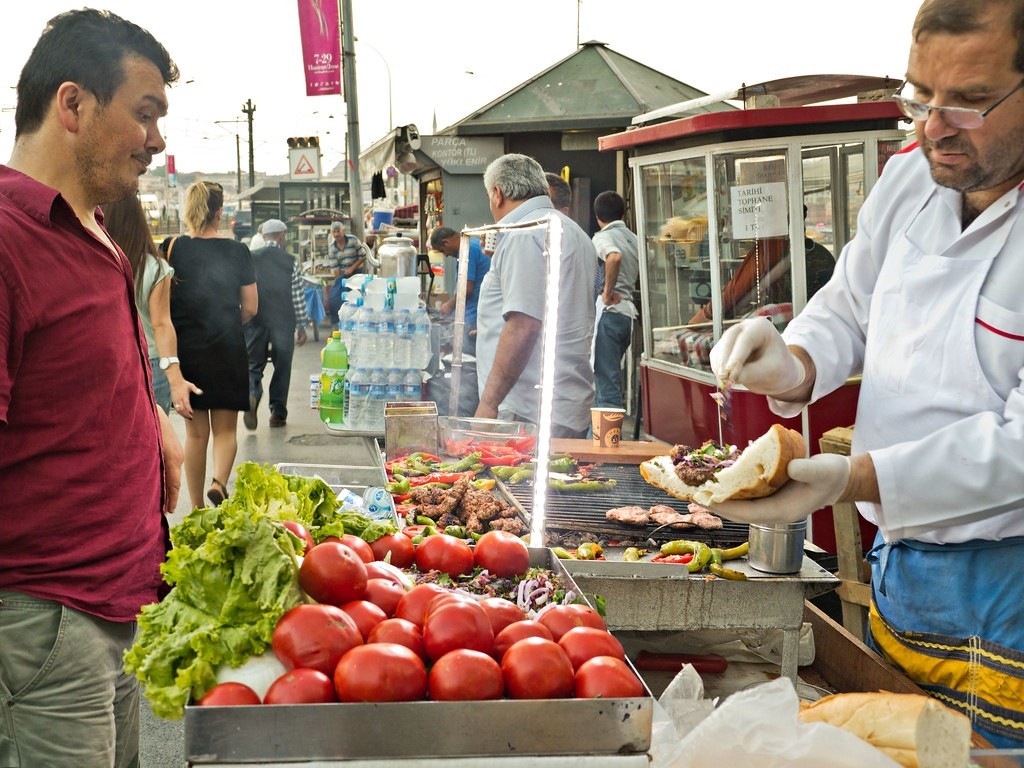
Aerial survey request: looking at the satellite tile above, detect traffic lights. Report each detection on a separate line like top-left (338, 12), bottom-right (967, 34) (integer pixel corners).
top-left (285, 137), bottom-right (320, 148)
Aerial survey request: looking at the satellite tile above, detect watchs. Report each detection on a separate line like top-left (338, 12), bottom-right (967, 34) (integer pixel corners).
top-left (160, 357), bottom-right (181, 371)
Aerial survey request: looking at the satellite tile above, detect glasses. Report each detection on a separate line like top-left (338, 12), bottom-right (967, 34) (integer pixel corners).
top-left (891, 80), bottom-right (1024, 130)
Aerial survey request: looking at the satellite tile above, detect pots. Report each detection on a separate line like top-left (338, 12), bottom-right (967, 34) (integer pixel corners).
top-left (440, 351), bottom-right (478, 374)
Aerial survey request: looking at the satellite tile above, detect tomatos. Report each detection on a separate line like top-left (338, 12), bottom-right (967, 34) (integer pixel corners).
top-left (190, 520), bottom-right (647, 706)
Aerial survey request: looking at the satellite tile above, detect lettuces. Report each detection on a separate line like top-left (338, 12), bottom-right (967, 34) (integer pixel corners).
top-left (119, 462), bottom-right (397, 723)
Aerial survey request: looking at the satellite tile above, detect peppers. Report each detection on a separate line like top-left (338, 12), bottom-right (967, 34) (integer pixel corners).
top-left (382, 430), bottom-right (750, 583)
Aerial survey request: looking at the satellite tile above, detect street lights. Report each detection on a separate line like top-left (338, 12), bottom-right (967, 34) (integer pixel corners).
top-left (203, 99), bottom-right (257, 211)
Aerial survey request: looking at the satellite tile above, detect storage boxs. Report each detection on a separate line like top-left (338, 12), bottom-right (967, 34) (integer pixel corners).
top-left (181, 547), bottom-right (652, 764)
top-left (384, 400), bottom-right (536, 461)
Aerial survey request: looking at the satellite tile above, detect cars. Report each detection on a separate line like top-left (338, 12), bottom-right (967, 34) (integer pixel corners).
top-left (228, 209), bottom-right (255, 242)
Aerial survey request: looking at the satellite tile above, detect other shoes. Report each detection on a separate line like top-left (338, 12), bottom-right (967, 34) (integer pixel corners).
top-left (244, 395), bottom-right (258, 429)
top-left (269, 416), bottom-right (285, 427)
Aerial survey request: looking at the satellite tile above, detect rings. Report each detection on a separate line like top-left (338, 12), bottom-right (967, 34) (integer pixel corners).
top-left (175, 404), bottom-right (180, 408)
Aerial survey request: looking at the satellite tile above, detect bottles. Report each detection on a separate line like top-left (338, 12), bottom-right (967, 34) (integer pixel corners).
top-left (318, 273), bottom-right (432, 430)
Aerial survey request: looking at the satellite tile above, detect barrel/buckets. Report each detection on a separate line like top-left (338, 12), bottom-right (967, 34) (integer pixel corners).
top-left (372, 209), bottom-right (393, 233)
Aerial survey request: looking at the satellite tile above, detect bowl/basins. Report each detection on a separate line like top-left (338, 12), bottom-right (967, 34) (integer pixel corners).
top-left (742, 679), bottom-right (833, 703)
top-left (438, 416), bottom-right (537, 459)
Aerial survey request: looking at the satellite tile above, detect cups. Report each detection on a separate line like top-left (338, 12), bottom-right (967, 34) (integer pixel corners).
top-left (589, 407), bottom-right (626, 448)
top-left (747, 518), bottom-right (806, 573)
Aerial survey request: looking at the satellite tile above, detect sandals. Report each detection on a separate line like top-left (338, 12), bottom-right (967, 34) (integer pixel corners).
top-left (207, 477), bottom-right (228, 506)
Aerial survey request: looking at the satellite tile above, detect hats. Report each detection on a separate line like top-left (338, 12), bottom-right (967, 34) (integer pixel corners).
top-left (261, 219), bottom-right (287, 236)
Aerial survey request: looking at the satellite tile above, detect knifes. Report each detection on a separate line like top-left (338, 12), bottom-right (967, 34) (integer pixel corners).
top-left (629, 649), bottom-right (727, 672)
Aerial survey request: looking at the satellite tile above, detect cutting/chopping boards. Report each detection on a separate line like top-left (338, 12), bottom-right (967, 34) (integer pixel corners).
top-left (551, 437), bottom-right (673, 465)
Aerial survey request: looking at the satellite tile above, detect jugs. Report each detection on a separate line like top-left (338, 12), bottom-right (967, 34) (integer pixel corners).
top-left (358, 231), bottom-right (437, 278)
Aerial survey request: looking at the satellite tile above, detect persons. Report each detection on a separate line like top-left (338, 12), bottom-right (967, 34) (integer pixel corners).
top-left (0, 7), bottom-right (176, 767)
top-left (97, 193), bottom-right (203, 420)
top-left (242, 218), bottom-right (307, 432)
top-left (544, 171), bottom-right (571, 216)
top-left (590, 190), bottom-right (641, 409)
top-left (250, 222), bottom-right (265, 249)
top-left (328, 221), bottom-right (368, 324)
top-left (707, 0), bottom-right (1024, 766)
top-left (474, 152), bottom-right (601, 438)
top-left (430, 226), bottom-right (493, 354)
top-left (775, 202), bottom-right (836, 303)
top-left (158, 178), bottom-right (259, 510)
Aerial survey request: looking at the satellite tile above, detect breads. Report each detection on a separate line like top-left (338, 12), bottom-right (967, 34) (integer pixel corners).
top-left (798, 689), bottom-right (971, 768)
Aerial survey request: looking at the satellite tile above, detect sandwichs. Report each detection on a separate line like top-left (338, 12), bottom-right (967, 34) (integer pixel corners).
top-left (641, 424), bottom-right (807, 504)
